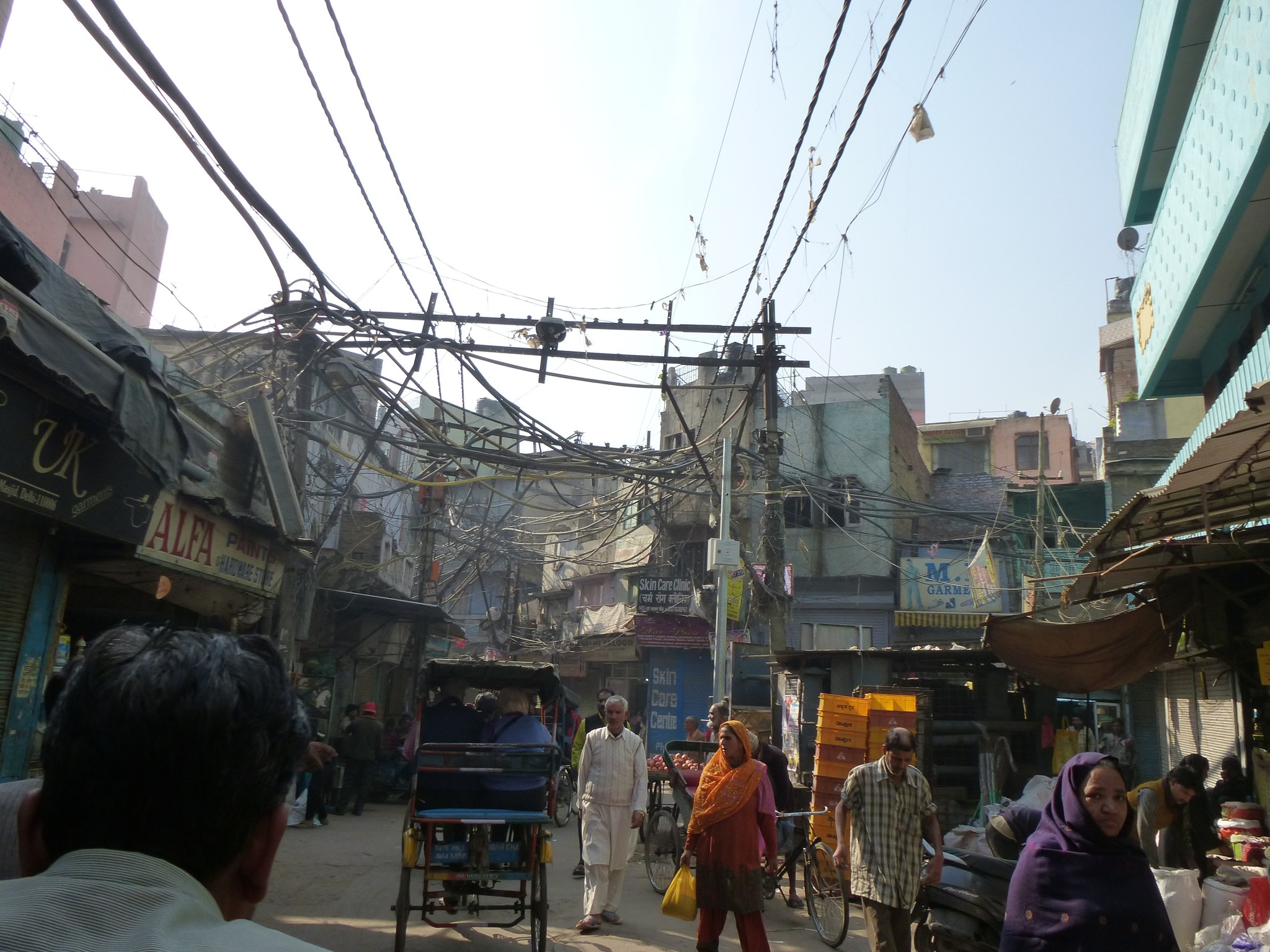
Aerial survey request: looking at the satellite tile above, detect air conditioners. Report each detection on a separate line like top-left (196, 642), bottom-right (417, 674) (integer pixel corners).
top-left (965, 427), bottom-right (986, 439)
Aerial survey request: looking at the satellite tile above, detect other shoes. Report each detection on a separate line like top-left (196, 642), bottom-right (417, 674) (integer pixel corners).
top-left (573, 864), bottom-right (585, 875)
top-left (327, 807), bottom-right (344, 814)
top-left (353, 808), bottom-right (361, 815)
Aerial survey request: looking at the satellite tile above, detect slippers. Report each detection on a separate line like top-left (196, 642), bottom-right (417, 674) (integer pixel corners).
top-left (790, 898), bottom-right (804, 908)
top-left (576, 916), bottom-right (602, 930)
top-left (321, 820), bottom-right (328, 824)
top-left (603, 912), bottom-right (622, 925)
top-left (295, 822), bottom-right (317, 829)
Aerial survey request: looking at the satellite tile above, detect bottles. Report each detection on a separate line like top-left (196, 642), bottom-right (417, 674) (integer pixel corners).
top-left (52, 629), bottom-right (71, 672)
top-left (72, 636), bottom-right (86, 661)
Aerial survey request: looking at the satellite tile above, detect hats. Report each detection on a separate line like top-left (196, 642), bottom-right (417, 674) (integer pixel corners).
top-left (747, 728), bottom-right (758, 751)
top-left (364, 702), bottom-right (376, 713)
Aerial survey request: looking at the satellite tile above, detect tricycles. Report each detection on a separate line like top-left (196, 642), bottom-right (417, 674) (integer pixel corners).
top-left (388, 658), bottom-right (580, 952)
top-left (642, 739), bottom-right (851, 949)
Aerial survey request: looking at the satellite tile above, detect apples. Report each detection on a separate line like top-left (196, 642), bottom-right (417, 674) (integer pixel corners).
top-left (645, 753), bottom-right (705, 771)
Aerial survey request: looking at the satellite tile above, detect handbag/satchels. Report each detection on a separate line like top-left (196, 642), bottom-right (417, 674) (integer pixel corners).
top-left (1051, 716), bottom-right (1078, 773)
top-left (661, 864), bottom-right (697, 922)
top-left (1042, 715), bottom-right (1055, 749)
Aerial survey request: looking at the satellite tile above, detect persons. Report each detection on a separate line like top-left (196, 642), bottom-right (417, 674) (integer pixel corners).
top-left (416, 678), bottom-right (574, 904)
top-left (684, 716), bottom-right (706, 764)
top-left (630, 712), bottom-right (646, 745)
top-left (680, 718), bottom-right (779, 952)
top-left (1127, 752), bottom-right (1254, 883)
top-left (833, 727), bottom-right (945, 952)
top-left (1000, 752), bottom-right (1181, 952)
top-left (575, 696), bottom-right (649, 930)
top-left (290, 681), bottom-right (414, 827)
top-left (0, 624), bottom-right (325, 952)
top-left (746, 731), bottom-right (805, 910)
top-left (1067, 716), bottom-right (1132, 774)
top-left (704, 703), bottom-right (729, 764)
top-left (571, 689), bottom-right (631, 879)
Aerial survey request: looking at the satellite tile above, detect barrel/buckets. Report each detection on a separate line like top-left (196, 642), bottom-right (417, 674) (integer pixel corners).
top-left (334, 764), bottom-right (345, 788)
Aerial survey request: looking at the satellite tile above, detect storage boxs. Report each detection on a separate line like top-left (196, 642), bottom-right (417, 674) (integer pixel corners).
top-left (808, 693), bottom-right (916, 900)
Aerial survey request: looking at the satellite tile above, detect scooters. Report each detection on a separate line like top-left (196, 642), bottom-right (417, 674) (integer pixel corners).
top-left (911, 839), bottom-right (1019, 952)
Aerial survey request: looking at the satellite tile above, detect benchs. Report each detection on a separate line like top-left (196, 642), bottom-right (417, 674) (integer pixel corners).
top-left (411, 742), bottom-right (562, 824)
